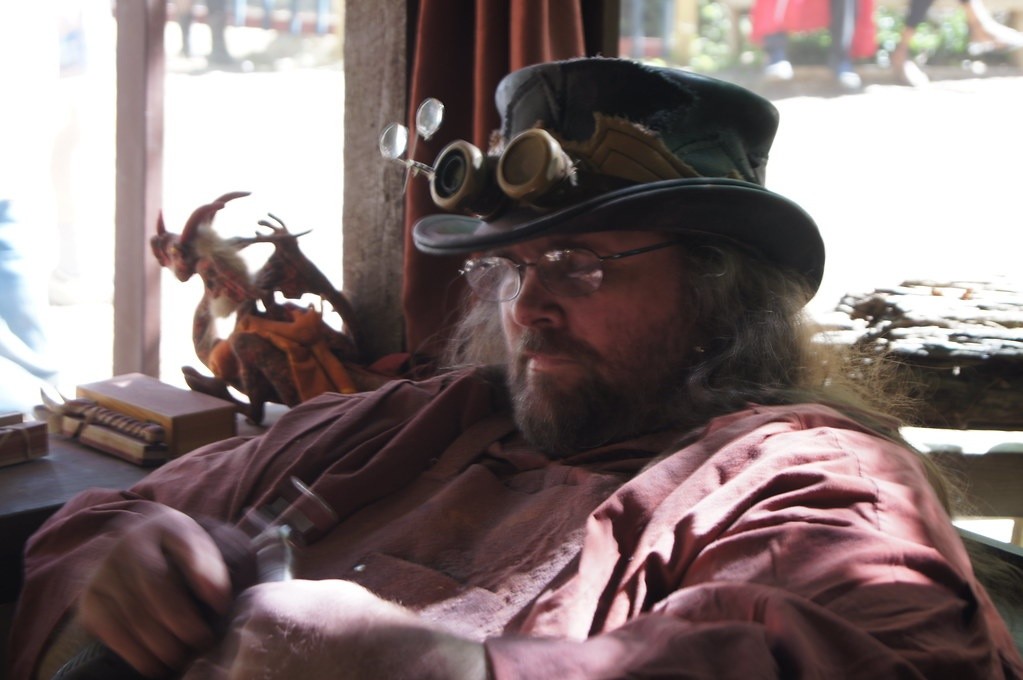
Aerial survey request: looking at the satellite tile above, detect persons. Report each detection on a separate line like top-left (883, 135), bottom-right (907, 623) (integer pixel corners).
top-left (891, 1), bottom-right (1023, 86)
top-left (749, 0), bottom-right (862, 91)
top-left (11, 58), bottom-right (1023, 680)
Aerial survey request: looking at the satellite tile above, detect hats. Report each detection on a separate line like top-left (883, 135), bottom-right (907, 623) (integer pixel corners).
top-left (412, 58), bottom-right (826, 318)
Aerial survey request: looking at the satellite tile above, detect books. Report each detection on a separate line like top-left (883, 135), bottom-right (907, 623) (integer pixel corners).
top-left (0, 373), bottom-right (238, 467)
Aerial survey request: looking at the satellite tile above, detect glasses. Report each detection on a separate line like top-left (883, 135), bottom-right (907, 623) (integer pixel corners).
top-left (458, 238), bottom-right (696, 302)
top-left (428, 130), bottom-right (584, 224)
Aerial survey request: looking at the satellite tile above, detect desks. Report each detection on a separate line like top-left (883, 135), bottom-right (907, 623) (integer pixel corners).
top-left (0, 403), bottom-right (295, 660)
